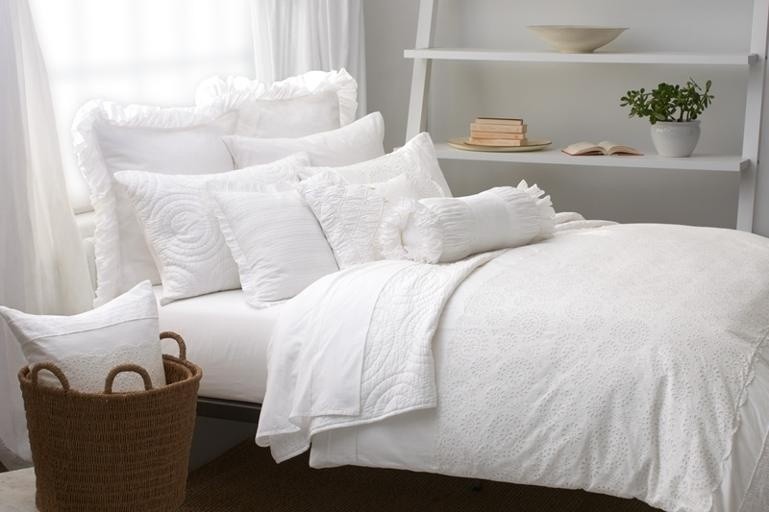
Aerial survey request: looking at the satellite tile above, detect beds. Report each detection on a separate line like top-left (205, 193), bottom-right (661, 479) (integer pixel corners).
top-left (33, 65), bottom-right (768, 510)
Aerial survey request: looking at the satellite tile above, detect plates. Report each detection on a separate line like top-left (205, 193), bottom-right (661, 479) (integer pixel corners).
top-left (447, 137), bottom-right (551, 152)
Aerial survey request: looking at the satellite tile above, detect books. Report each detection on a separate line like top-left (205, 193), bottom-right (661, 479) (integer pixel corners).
top-left (562, 139), bottom-right (643, 155)
top-left (466, 116), bottom-right (528, 147)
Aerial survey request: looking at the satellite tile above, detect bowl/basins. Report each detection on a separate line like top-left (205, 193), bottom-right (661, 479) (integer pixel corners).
top-left (525, 25), bottom-right (629, 53)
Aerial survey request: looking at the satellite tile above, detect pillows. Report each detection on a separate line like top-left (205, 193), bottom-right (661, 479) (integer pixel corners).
top-left (378, 177), bottom-right (560, 264)
top-left (109, 145), bottom-right (314, 308)
top-left (1, 278), bottom-right (168, 392)
top-left (192, 65), bottom-right (362, 168)
top-left (204, 186), bottom-right (342, 308)
top-left (67, 88), bottom-right (263, 314)
top-left (217, 107), bottom-right (388, 168)
top-left (297, 129), bottom-right (455, 270)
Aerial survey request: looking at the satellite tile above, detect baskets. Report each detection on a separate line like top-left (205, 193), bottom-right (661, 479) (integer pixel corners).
top-left (18, 332), bottom-right (203, 512)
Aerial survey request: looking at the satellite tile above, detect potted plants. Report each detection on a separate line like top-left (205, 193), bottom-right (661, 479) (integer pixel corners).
top-left (616, 75), bottom-right (716, 156)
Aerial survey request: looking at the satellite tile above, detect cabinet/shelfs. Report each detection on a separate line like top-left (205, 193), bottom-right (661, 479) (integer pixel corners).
top-left (400, 1), bottom-right (767, 240)
